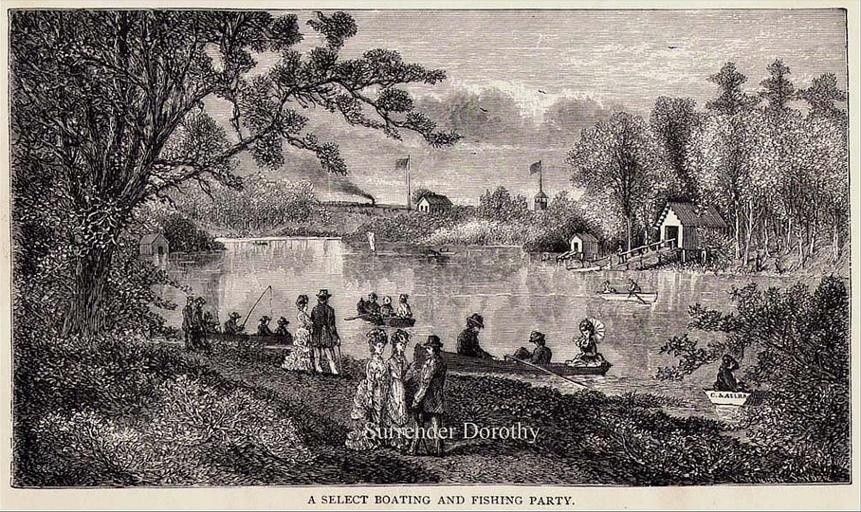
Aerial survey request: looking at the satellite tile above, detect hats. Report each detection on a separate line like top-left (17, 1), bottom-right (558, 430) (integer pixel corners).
top-left (468, 314), bottom-right (484, 327)
top-left (529, 331), bottom-right (545, 342)
top-left (316, 290), bottom-right (332, 297)
top-left (229, 312), bottom-right (240, 318)
top-left (277, 318), bottom-right (289, 324)
top-left (259, 316), bottom-right (272, 321)
top-left (424, 335), bottom-right (443, 347)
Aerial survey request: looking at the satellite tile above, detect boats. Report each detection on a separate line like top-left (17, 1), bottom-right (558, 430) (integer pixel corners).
top-left (702, 384), bottom-right (764, 407)
top-left (200, 328), bottom-right (296, 347)
top-left (358, 297), bottom-right (416, 329)
top-left (597, 289), bottom-right (660, 304)
top-left (412, 342), bottom-right (613, 379)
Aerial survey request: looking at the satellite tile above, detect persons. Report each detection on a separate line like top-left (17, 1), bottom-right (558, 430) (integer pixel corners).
top-left (600, 279), bottom-right (617, 293)
top-left (342, 328), bottom-right (395, 453)
top-left (311, 290), bottom-right (341, 377)
top-left (183, 288), bottom-right (293, 354)
top-left (410, 334), bottom-right (447, 456)
top-left (280, 295), bottom-right (314, 373)
top-left (627, 278), bottom-right (642, 293)
top-left (716, 353), bottom-right (744, 392)
top-left (564, 318), bottom-right (607, 368)
top-left (368, 292), bottom-right (414, 320)
top-left (455, 313), bottom-right (499, 361)
top-left (513, 331), bottom-right (554, 366)
top-left (385, 328), bottom-right (414, 450)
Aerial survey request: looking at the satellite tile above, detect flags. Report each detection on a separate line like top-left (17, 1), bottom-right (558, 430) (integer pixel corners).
top-left (529, 160), bottom-right (543, 175)
top-left (396, 158), bottom-right (410, 172)
